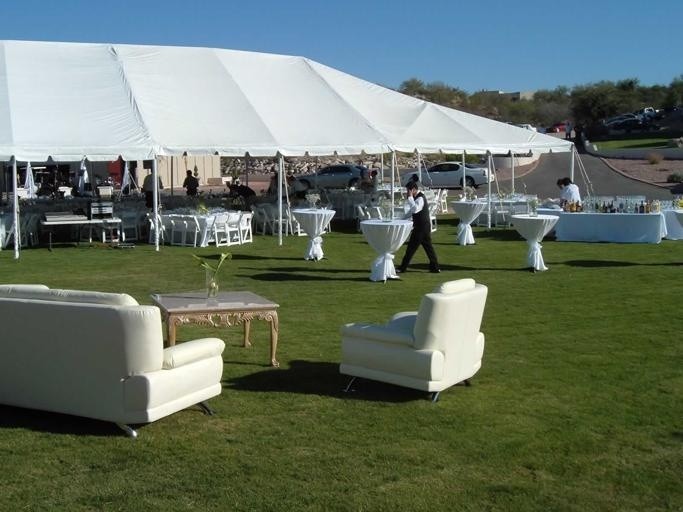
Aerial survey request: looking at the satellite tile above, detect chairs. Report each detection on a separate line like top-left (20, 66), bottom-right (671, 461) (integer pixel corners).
top-left (252, 187), bottom-right (582, 239)
top-left (340, 278), bottom-right (488, 402)
top-left (0, 188), bottom-right (255, 250)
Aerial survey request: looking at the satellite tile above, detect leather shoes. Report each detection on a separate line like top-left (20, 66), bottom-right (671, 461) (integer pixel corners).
top-left (430, 268), bottom-right (441, 273)
top-left (396, 268), bottom-right (407, 273)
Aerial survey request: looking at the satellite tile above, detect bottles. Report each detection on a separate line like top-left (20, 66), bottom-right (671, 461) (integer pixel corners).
top-left (563, 199), bottom-right (660, 214)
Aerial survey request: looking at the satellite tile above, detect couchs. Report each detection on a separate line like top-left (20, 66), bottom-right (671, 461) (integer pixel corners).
top-left (0, 283), bottom-right (225, 437)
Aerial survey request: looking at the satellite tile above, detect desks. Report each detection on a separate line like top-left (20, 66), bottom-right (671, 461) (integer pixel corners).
top-left (657, 199), bottom-right (683, 240)
top-left (509, 214), bottom-right (560, 272)
top-left (556, 210), bottom-right (664, 243)
top-left (293, 209), bottom-right (337, 261)
top-left (360, 219), bottom-right (413, 283)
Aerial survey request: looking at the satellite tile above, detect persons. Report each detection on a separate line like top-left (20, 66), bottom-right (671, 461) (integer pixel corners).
top-left (143, 167), bottom-right (164, 212)
top-left (359, 170), bottom-right (379, 190)
top-left (395, 181), bottom-right (441, 273)
top-left (267, 171), bottom-right (307, 201)
top-left (183, 170), bottom-right (199, 197)
top-left (566, 122), bottom-right (572, 140)
top-left (408, 174), bottom-right (424, 187)
top-left (557, 177), bottom-right (581, 206)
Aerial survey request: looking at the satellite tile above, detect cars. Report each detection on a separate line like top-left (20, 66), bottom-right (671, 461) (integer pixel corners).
top-left (398, 161), bottom-right (494, 191)
top-left (288, 162), bottom-right (381, 191)
top-left (221, 156), bottom-right (436, 176)
top-left (504, 104), bottom-right (679, 133)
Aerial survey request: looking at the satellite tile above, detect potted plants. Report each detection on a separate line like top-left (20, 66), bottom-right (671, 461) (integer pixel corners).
top-left (190, 253), bottom-right (233, 298)
